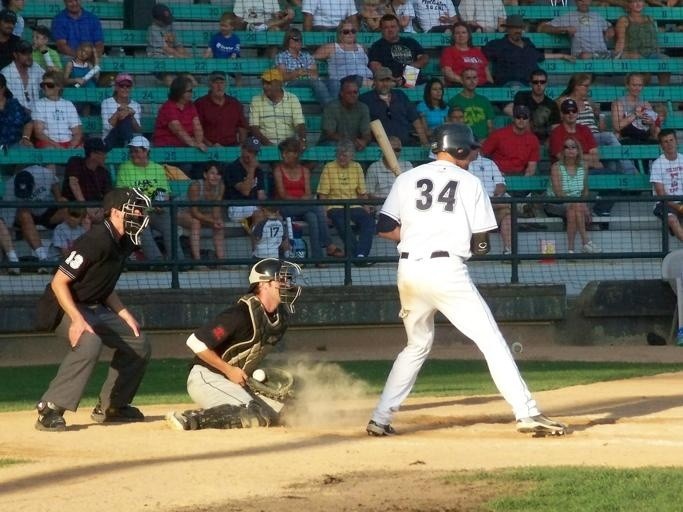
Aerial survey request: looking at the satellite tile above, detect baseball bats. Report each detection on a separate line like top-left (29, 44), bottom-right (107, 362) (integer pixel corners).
top-left (369, 119), bottom-right (403, 178)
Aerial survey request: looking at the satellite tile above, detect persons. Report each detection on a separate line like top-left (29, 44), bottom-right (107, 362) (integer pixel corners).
top-left (169, 259), bottom-right (302, 431)
top-left (0, 0), bottom-right (682, 274)
top-left (367, 124), bottom-right (568, 436)
top-left (30, 187), bottom-right (151, 431)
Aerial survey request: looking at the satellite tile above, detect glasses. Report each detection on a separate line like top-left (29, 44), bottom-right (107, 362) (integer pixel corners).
top-left (392, 148), bottom-right (401, 152)
top-left (531, 80), bottom-right (546, 85)
top-left (514, 115), bottom-right (529, 120)
top-left (560, 108), bottom-right (578, 114)
top-left (39, 82), bottom-right (58, 89)
top-left (341, 28), bottom-right (357, 35)
top-left (287, 36), bottom-right (302, 42)
top-left (563, 144), bottom-right (577, 149)
top-left (117, 84), bottom-right (133, 89)
top-left (17, 48), bottom-right (33, 56)
top-left (186, 88), bottom-right (193, 93)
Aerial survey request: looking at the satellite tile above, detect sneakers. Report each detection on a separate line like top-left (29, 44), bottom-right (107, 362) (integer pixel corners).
top-left (36, 401), bottom-right (66, 432)
top-left (166, 411), bottom-right (189, 430)
top-left (92, 403), bottom-right (144, 423)
top-left (366, 420), bottom-right (396, 436)
top-left (517, 415), bottom-right (567, 436)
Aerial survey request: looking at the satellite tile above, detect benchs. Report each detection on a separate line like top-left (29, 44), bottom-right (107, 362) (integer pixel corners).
top-left (0, 0), bottom-right (683, 255)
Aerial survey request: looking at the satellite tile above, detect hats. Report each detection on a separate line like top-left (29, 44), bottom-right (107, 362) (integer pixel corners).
top-left (29, 24), bottom-right (51, 38)
top-left (500, 14), bottom-right (529, 28)
top-left (0, 9), bottom-right (17, 24)
top-left (374, 67), bottom-right (394, 81)
top-left (84, 136), bottom-right (113, 155)
top-left (257, 69), bottom-right (283, 81)
top-left (153, 3), bottom-right (176, 25)
top-left (14, 171), bottom-right (35, 197)
top-left (115, 75), bottom-right (133, 85)
top-left (513, 106), bottom-right (530, 118)
top-left (129, 136), bottom-right (151, 150)
top-left (244, 137), bottom-right (261, 152)
top-left (561, 99), bottom-right (577, 111)
top-left (11, 40), bottom-right (32, 53)
top-left (209, 70), bottom-right (226, 82)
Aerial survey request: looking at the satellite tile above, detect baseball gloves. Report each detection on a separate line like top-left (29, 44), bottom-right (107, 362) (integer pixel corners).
top-left (247, 367), bottom-right (295, 402)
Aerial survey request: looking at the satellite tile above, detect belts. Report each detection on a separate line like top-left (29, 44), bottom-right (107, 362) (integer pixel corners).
top-left (400, 251), bottom-right (452, 257)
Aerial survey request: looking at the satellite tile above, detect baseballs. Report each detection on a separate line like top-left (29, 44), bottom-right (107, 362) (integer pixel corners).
top-left (252, 369), bottom-right (266, 383)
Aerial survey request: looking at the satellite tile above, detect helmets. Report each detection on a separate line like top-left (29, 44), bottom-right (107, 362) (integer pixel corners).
top-left (431, 121), bottom-right (482, 160)
top-left (102, 190), bottom-right (150, 246)
top-left (249, 258), bottom-right (302, 315)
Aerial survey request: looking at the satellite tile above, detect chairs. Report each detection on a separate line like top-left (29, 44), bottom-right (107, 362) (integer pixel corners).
top-left (661, 249), bottom-right (683, 345)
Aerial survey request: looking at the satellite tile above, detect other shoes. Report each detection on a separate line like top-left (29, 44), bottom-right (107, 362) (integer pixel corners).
top-left (144, 259), bottom-right (236, 272)
top-left (313, 248), bottom-right (376, 268)
top-left (582, 242), bottom-right (602, 254)
top-left (37, 261), bottom-right (48, 274)
top-left (502, 250), bottom-right (520, 264)
top-left (8, 262), bottom-right (20, 275)
